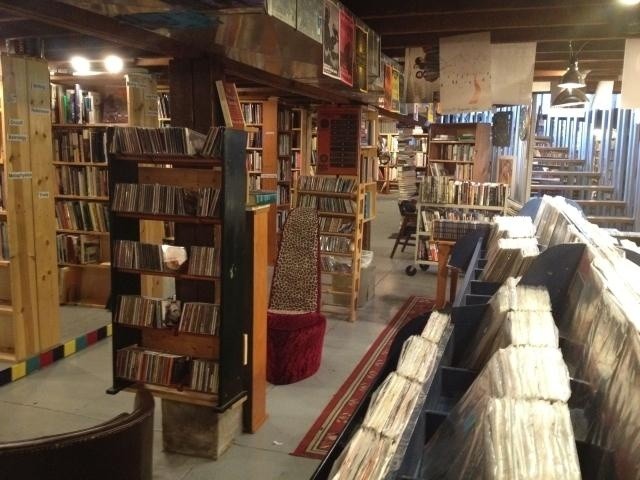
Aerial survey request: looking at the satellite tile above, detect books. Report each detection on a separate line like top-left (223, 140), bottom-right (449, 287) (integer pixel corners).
top-left (1, 81), bottom-right (639, 478)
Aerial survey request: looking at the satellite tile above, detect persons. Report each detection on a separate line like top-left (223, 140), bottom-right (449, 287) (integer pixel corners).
top-left (412, 44), bottom-right (440, 82)
